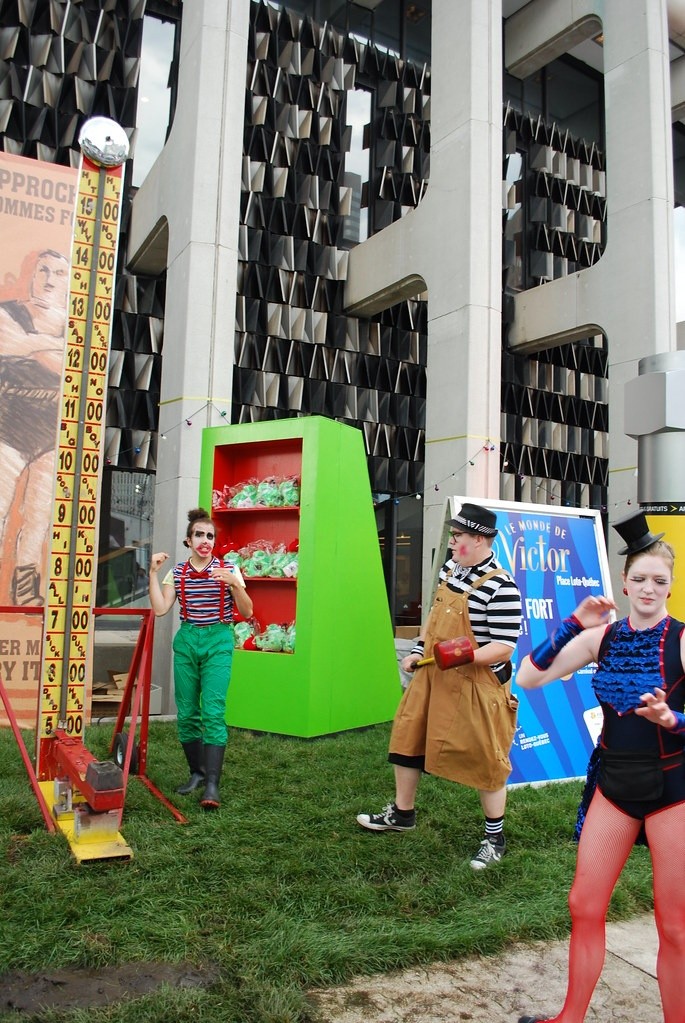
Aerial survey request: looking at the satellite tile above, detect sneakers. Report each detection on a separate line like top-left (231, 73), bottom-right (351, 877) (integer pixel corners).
top-left (356, 801), bottom-right (416, 831)
top-left (469, 833), bottom-right (507, 871)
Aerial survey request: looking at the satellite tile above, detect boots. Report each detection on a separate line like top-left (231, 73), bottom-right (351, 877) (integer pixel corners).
top-left (177, 738), bottom-right (206, 795)
top-left (199, 743), bottom-right (225, 808)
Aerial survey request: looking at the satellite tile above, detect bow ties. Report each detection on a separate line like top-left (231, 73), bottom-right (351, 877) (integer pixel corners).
top-left (189, 571), bottom-right (208, 579)
top-left (452, 563), bottom-right (472, 580)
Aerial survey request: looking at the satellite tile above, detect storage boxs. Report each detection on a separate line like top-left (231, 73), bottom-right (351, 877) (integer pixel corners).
top-left (396, 625), bottom-right (422, 639)
top-left (92, 669), bottom-right (139, 718)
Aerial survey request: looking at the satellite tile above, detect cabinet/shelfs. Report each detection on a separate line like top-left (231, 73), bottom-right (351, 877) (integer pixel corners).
top-left (212, 505), bottom-right (298, 583)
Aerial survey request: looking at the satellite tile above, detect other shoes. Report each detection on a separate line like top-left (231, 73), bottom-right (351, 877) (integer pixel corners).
top-left (518, 1013), bottom-right (549, 1023)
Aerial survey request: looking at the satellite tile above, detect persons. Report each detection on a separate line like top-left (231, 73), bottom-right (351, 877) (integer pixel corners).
top-left (515, 508), bottom-right (685, 1023)
top-left (149, 507), bottom-right (253, 809)
top-left (355, 503), bottom-right (520, 870)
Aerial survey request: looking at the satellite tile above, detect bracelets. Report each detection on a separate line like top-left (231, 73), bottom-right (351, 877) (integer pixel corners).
top-left (411, 640), bottom-right (425, 657)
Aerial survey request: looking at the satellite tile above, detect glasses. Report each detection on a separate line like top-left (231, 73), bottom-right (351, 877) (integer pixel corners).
top-left (447, 530), bottom-right (470, 542)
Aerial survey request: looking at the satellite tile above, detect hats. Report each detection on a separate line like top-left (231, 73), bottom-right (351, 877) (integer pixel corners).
top-left (444, 503), bottom-right (498, 538)
